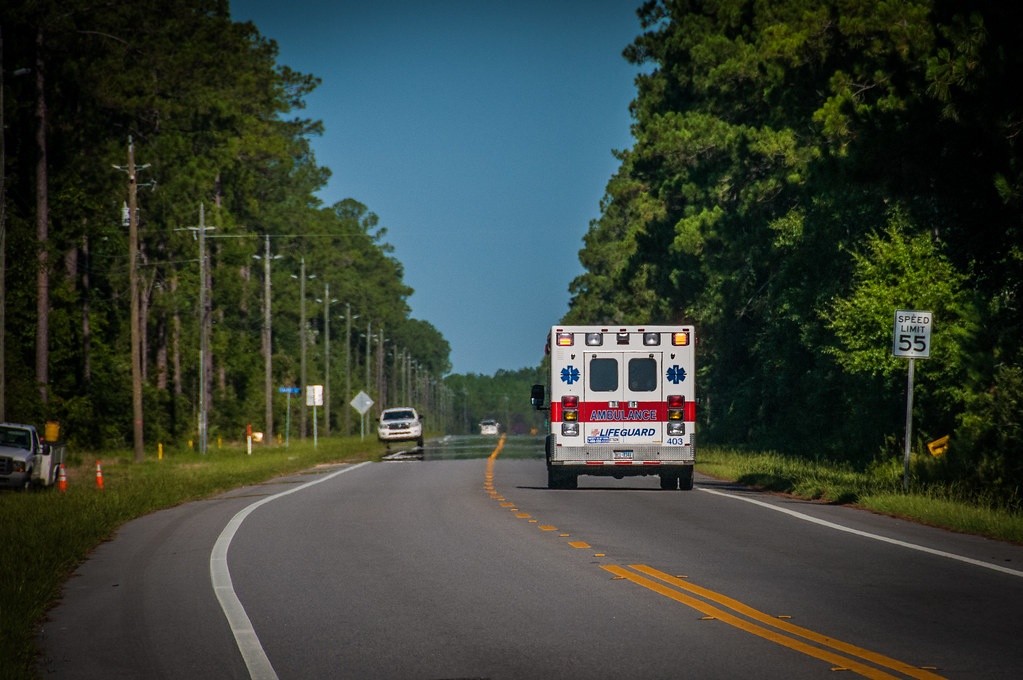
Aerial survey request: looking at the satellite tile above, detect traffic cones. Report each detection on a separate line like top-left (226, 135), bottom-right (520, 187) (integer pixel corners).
top-left (94, 459), bottom-right (106, 490)
top-left (57, 463), bottom-right (69, 494)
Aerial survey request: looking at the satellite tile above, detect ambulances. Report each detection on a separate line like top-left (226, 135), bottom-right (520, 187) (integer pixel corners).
top-left (528, 324), bottom-right (700, 490)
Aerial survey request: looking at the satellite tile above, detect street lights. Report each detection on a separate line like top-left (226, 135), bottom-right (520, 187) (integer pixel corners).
top-left (252, 234), bottom-right (285, 453)
top-left (110, 133), bottom-right (153, 462)
top-left (186, 202), bottom-right (217, 457)
top-left (359, 321), bottom-right (377, 436)
top-left (388, 344), bottom-right (404, 408)
top-left (373, 327), bottom-right (390, 413)
top-left (314, 283), bottom-right (340, 438)
top-left (290, 258), bottom-right (317, 445)
top-left (337, 301), bottom-right (363, 437)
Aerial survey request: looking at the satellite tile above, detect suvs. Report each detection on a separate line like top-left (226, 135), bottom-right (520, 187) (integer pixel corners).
top-left (374, 407), bottom-right (426, 448)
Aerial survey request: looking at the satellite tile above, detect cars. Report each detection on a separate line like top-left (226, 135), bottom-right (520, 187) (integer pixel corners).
top-left (0, 421), bottom-right (50, 494)
top-left (478, 420), bottom-right (501, 436)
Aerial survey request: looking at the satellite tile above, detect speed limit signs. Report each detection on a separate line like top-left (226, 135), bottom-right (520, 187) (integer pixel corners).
top-left (893, 309), bottom-right (932, 360)
